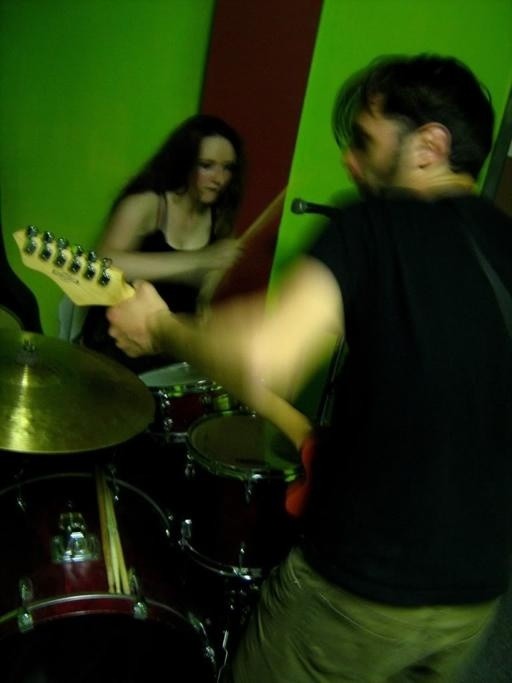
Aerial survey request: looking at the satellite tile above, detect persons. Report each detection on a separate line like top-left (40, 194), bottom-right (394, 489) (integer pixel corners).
top-left (71, 113), bottom-right (247, 375)
top-left (105, 58), bottom-right (512, 683)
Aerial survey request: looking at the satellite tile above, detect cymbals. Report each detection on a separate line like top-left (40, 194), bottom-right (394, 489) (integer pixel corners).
top-left (0, 326), bottom-right (157, 453)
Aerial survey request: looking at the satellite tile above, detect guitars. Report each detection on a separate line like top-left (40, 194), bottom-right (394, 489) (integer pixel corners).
top-left (13, 226), bottom-right (320, 516)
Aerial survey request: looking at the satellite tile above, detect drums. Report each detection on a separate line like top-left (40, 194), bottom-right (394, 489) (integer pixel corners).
top-left (0, 471), bottom-right (216, 683)
top-left (171, 410), bottom-right (304, 578)
top-left (142, 364), bottom-right (234, 453)
